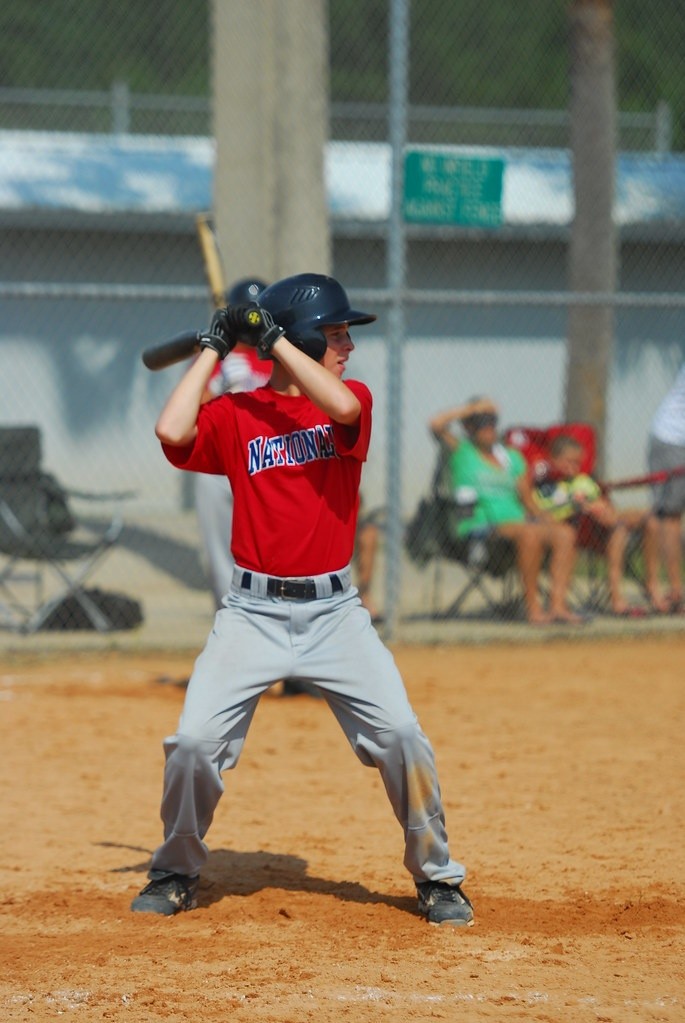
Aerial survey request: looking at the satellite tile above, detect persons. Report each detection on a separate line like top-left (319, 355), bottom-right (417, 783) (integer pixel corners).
top-left (133, 276), bottom-right (476, 928)
top-left (532, 432), bottom-right (673, 617)
top-left (648, 365), bottom-right (684, 617)
top-left (428, 395), bottom-right (586, 624)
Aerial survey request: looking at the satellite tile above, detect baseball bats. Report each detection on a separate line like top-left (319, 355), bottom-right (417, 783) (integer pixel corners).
top-left (142, 330), bottom-right (203, 371)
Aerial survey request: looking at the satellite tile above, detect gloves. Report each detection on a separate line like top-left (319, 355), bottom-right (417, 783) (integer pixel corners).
top-left (217, 301), bottom-right (286, 361)
top-left (199, 308), bottom-right (240, 360)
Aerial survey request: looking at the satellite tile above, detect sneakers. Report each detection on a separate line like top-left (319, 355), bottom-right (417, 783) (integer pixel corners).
top-left (414, 880), bottom-right (476, 927)
top-left (129, 871), bottom-right (199, 916)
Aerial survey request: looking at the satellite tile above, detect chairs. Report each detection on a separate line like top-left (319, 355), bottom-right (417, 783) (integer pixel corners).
top-left (511, 423), bottom-right (646, 614)
top-left (0, 426), bottom-right (124, 632)
top-left (433, 464), bottom-right (518, 625)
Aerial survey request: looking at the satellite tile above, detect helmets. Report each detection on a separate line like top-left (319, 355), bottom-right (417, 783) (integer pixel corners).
top-left (258, 271), bottom-right (378, 362)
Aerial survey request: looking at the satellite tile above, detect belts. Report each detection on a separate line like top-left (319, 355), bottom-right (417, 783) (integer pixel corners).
top-left (240, 570), bottom-right (344, 602)
